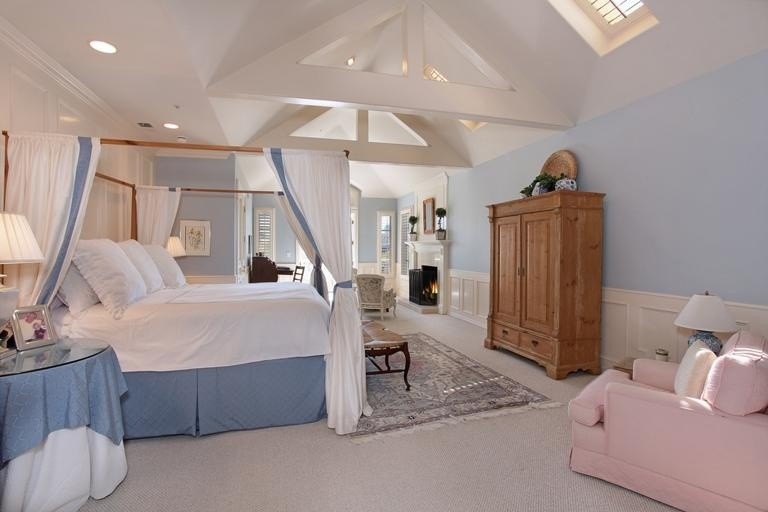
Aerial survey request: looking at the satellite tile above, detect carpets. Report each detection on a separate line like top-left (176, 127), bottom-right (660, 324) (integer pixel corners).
top-left (343, 325), bottom-right (565, 450)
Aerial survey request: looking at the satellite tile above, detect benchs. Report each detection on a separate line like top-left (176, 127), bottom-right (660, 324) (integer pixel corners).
top-left (359, 320), bottom-right (412, 392)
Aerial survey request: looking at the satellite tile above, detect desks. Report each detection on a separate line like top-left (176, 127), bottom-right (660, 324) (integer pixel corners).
top-left (6, 334), bottom-right (131, 511)
top-left (251, 255), bottom-right (294, 282)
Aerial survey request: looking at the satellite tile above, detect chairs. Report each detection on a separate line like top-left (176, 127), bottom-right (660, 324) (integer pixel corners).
top-left (289, 265), bottom-right (305, 284)
top-left (353, 273), bottom-right (398, 322)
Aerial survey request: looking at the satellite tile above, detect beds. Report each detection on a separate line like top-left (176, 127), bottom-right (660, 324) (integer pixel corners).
top-left (1, 127), bottom-right (370, 440)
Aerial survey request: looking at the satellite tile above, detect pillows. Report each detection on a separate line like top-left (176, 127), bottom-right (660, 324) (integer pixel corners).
top-left (142, 242), bottom-right (187, 291)
top-left (69, 237), bottom-right (148, 321)
top-left (115, 239), bottom-right (165, 295)
top-left (671, 339), bottom-right (718, 400)
top-left (53, 249), bottom-right (101, 320)
top-left (46, 294), bottom-right (63, 312)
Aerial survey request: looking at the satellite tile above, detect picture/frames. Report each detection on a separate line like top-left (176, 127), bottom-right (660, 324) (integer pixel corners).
top-left (177, 219), bottom-right (212, 257)
top-left (9, 304), bottom-right (59, 352)
top-left (422, 199), bottom-right (435, 234)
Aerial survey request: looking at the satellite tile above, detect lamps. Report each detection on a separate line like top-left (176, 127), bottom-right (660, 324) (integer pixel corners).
top-left (166, 234), bottom-right (187, 260)
top-left (673, 288), bottom-right (741, 358)
top-left (0, 209), bottom-right (45, 361)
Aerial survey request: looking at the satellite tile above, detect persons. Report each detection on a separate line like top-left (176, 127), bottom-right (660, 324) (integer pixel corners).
top-left (40, 320), bottom-right (47, 332)
top-left (32, 320), bottom-right (48, 340)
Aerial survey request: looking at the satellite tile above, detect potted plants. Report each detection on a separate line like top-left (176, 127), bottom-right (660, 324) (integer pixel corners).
top-left (516, 173), bottom-right (580, 197)
top-left (435, 208), bottom-right (448, 240)
top-left (408, 215), bottom-right (418, 242)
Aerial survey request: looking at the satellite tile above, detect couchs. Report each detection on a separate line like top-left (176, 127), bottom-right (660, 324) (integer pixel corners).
top-left (564, 331), bottom-right (768, 511)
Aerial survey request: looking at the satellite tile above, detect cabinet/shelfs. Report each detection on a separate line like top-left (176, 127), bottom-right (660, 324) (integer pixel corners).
top-left (476, 190), bottom-right (607, 382)
